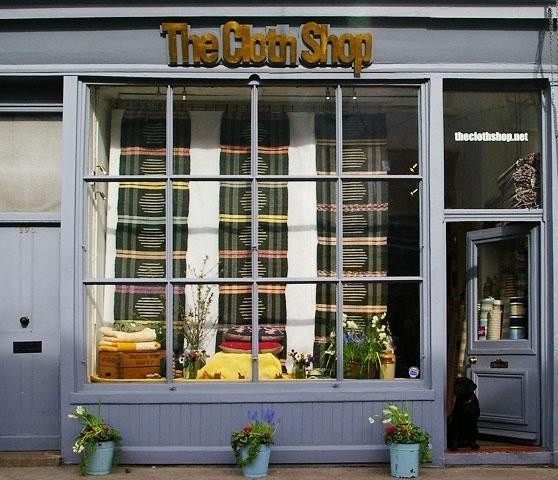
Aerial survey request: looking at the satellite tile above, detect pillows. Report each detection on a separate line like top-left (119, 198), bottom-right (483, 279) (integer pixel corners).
top-left (219, 339), bottom-right (282, 354)
top-left (223, 323), bottom-right (286, 340)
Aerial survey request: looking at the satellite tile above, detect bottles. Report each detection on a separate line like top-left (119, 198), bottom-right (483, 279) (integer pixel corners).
top-left (378, 349), bottom-right (397, 381)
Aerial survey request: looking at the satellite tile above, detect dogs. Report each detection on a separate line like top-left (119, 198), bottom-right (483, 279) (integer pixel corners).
top-left (447, 377), bottom-right (481, 451)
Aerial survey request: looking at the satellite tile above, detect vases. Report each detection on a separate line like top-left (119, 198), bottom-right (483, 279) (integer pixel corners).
top-left (240, 445), bottom-right (270, 477)
top-left (184, 346), bottom-right (209, 380)
top-left (295, 365), bottom-right (306, 378)
top-left (391, 442), bottom-right (421, 478)
top-left (379, 350), bottom-right (395, 379)
top-left (84, 441), bottom-right (116, 475)
top-left (349, 359), bottom-right (374, 378)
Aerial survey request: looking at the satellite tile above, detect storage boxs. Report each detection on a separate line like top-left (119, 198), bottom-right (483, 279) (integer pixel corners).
top-left (98, 349), bottom-right (165, 379)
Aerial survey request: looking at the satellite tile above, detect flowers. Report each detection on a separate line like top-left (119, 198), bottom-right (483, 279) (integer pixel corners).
top-left (369, 400), bottom-right (433, 462)
top-left (66, 406), bottom-right (121, 474)
top-left (289, 349), bottom-right (315, 366)
top-left (152, 253), bottom-right (217, 364)
top-left (371, 313), bottom-right (394, 350)
top-left (323, 312), bottom-right (391, 378)
top-left (230, 420), bottom-right (279, 467)
